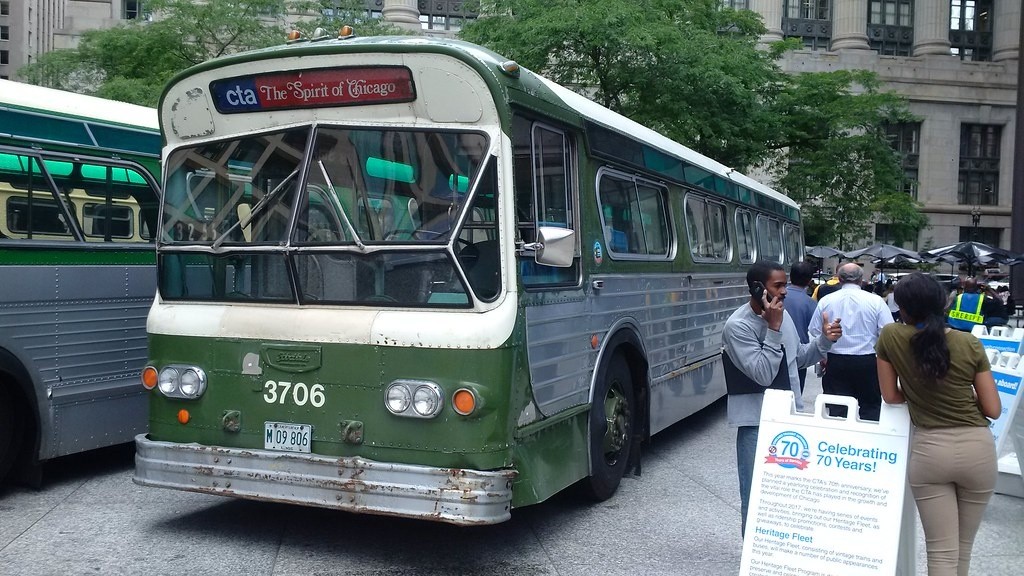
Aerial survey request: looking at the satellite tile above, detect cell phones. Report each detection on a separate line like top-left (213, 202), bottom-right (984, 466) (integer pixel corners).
top-left (749, 281), bottom-right (773, 312)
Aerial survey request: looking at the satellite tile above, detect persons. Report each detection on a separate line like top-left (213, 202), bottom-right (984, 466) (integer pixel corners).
top-left (873, 273), bottom-right (1002, 576)
top-left (784, 261), bottom-right (818, 395)
top-left (720, 260), bottom-right (843, 542)
top-left (807, 260), bottom-right (1014, 334)
top-left (807, 263), bottom-right (895, 421)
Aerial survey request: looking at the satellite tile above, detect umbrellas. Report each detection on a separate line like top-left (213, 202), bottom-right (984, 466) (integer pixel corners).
top-left (802, 240), bottom-right (1024, 283)
